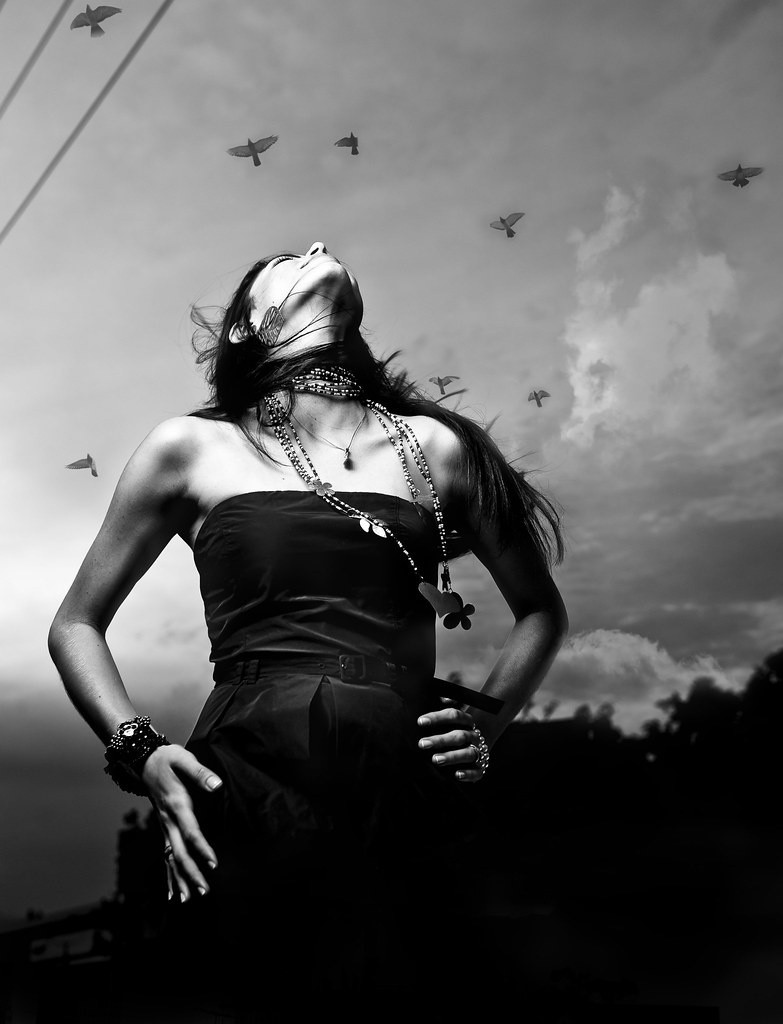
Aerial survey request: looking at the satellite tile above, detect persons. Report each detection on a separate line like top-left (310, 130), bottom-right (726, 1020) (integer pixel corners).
top-left (42, 240), bottom-right (569, 1024)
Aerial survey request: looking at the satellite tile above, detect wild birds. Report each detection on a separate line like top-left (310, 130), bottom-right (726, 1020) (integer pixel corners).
top-left (429, 375), bottom-right (460, 395)
top-left (717, 163), bottom-right (764, 188)
top-left (528, 390), bottom-right (551, 408)
top-left (490, 211), bottom-right (525, 239)
top-left (65, 453), bottom-right (98, 477)
top-left (69, 3), bottom-right (122, 39)
top-left (335, 131), bottom-right (359, 155)
top-left (225, 135), bottom-right (279, 167)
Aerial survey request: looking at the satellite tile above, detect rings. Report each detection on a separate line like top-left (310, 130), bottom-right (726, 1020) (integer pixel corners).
top-left (470, 744), bottom-right (481, 765)
top-left (164, 845), bottom-right (173, 862)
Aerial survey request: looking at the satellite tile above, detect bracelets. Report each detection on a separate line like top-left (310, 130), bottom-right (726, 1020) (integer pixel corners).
top-left (473, 723), bottom-right (490, 775)
top-left (103, 714), bottom-right (171, 798)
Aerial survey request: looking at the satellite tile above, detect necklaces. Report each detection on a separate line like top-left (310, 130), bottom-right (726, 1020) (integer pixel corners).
top-left (263, 365), bottom-right (475, 631)
